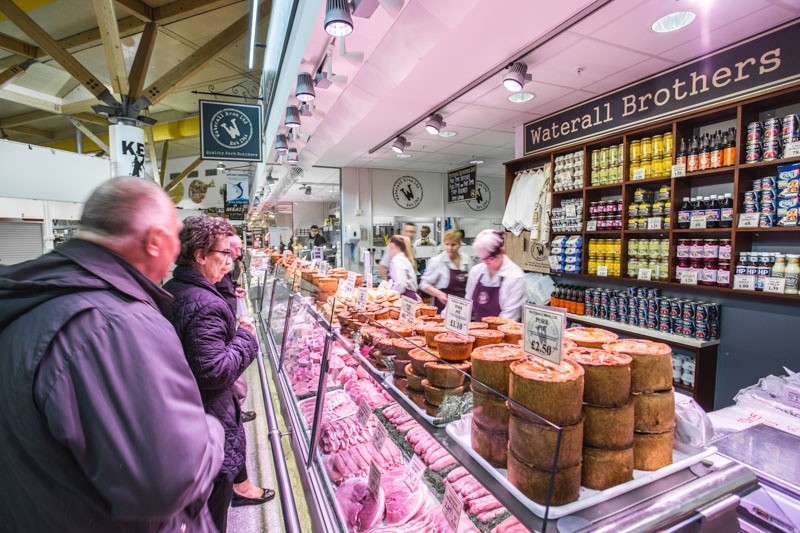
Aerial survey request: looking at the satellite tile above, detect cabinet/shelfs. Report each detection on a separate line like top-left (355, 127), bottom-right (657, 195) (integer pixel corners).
top-left (567, 315), bottom-right (721, 415)
top-left (503, 80), bottom-right (800, 300)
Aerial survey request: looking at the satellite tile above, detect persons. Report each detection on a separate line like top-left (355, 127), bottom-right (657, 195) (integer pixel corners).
top-left (464, 229), bottom-right (526, 322)
top-left (387, 235), bottom-right (423, 302)
top-left (161, 218), bottom-right (258, 533)
top-left (307, 225), bottom-right (326, 250)
top-left (1, 175), bottom-right (227, 533)
top-left (213, 235), bottom-right (257, 422)
top-left (378, 222), bottom-right (416, 280)
top-left (420, 230), bottom-right (472, 314)
top-left (414, 225), bottom-right (437, 246)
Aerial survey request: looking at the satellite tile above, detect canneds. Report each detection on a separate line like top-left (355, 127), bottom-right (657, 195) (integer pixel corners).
top-left (746, 114), bottom-right (800, 164)
top-left (591, 143), bottom-right (623, 184)
top-left (587, 199), bottom-right (622, 231)
top-left (626, 239), bottom-right (669, 279)
top-left (628, 132), bottom-right (674, 181)
top-left (583, 286), bottom-right (720, 342)
top-left (674, 238), bottom-right (731, 288)
top-left (742, 176), bottom-right (776, 227)
top-left (627, 183), bottom-right (671, 229)
top-left (587, 239), bottom-right (621, 277)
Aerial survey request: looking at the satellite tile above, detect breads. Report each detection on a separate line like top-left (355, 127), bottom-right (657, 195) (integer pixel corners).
top-left (271, 252), bottom-right (676, 508)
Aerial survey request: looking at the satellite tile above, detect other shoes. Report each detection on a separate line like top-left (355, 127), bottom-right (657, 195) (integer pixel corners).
top-left (241, 411), bottom-right (255, 422)
top-left (231, 488), bottom-right (276, 507)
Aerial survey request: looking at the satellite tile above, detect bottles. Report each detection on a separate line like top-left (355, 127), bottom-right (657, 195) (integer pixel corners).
top-left (677, 193), bottom-right (733, 229)
top-left (674, 126), bottom-right (737, 172)
top-left (550, 283), bottom-right (588, 315)
top-left (771, 253), bottom-right (800, 294)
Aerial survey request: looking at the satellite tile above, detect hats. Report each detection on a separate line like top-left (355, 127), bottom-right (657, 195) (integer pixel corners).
top-left (473, 229), bottom-right (506, 258)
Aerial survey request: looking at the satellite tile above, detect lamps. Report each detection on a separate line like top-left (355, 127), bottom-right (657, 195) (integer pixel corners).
top-left (217, 160), bottom-right (226, 170)
top-left (503, 59), bottom-right (532, 93)
top-left (275, 134), bottom-right (295, 151)
top-left (286, 148), bottom-right (298, 162)
top-left (295, 72), bottom-right (334, 102)
top-left (392, 134), bottom-right (411, 154)
top-left (324, 1), bottom-right (353, 38)
top-left (425, 112), bottom-right (446, 136)
top-left (285, 104), bottom-right (311, 128)
top-left (304, 184), bottom-right (312, 195)
top-left (266, 172), bottom-right (275, 185)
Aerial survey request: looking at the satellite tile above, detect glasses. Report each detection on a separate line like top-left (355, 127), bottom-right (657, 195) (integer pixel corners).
top-left (209, 249), bottom-right (231, 257)
